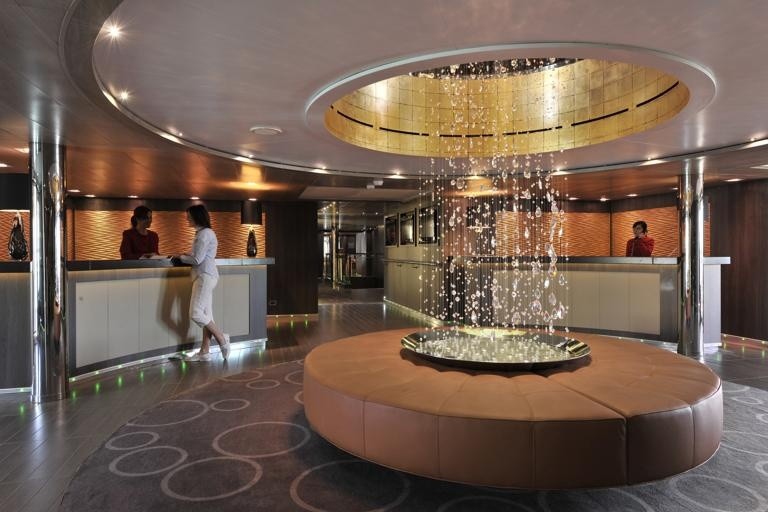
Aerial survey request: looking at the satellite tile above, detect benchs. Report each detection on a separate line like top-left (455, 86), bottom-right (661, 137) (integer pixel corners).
top-left (304, 329), bottom-right (724, 490)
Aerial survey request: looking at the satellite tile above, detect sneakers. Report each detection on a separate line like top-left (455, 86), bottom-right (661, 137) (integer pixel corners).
top-left (184, 351), bottom-right (212, 363)
top-left (221, 332), bottom-right (230, 360)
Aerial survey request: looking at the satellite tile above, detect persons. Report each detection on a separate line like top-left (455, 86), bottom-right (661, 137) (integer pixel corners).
top-left (118, 205), bottom-right (160, 261)
top-left (164, 200), bottom-right (231, 364)
top-left (625, 219), bottom-right (655, 256)
